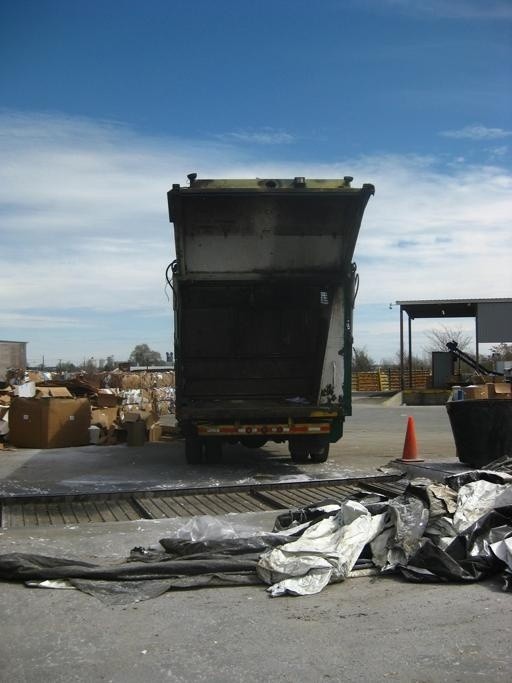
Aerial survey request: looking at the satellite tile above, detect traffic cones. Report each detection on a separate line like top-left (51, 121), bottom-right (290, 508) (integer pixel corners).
top-left (395, 415), bottom-right (424, 461)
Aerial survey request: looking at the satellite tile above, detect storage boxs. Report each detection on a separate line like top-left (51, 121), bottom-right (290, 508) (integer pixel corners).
top-left (9, 397), bottom-right (91, 450)
top-left (464, 384), bottom-right (488, 400)
top-left (96, 392), bottom-right (117, 406)
top-left (31, 387), bottom-right (72, 399)
top-left (485, 383), bottom-right (511, 400)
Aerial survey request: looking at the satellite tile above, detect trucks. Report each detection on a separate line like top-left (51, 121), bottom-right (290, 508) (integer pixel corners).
top-left (165, 173), bottom-right (376, 463)
